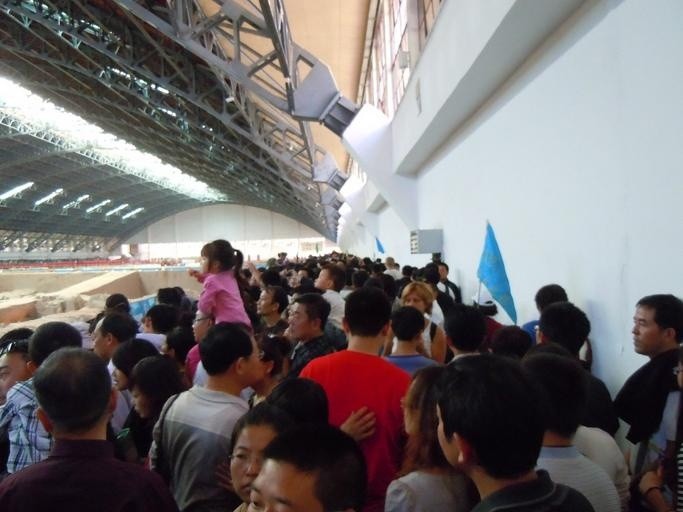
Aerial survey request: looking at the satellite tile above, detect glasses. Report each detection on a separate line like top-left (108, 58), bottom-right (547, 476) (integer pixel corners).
top-left (191, 315), bottom-right (212, 325)
top-left (245, 350), bottom-right (264, 360)
top-left (228, 452), bottom-right (261, 468)
top-left (0, 341), bottom-right (28, 357)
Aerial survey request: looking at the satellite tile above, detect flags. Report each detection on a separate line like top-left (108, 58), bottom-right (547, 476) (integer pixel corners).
top-left (475, 218), bottom-right (518, 326)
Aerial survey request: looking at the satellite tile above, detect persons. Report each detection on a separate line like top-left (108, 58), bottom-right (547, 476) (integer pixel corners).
top-left (188, 239), bottom-right (256, 334)
top-left (0, 250), bottom-right (682, 512)
